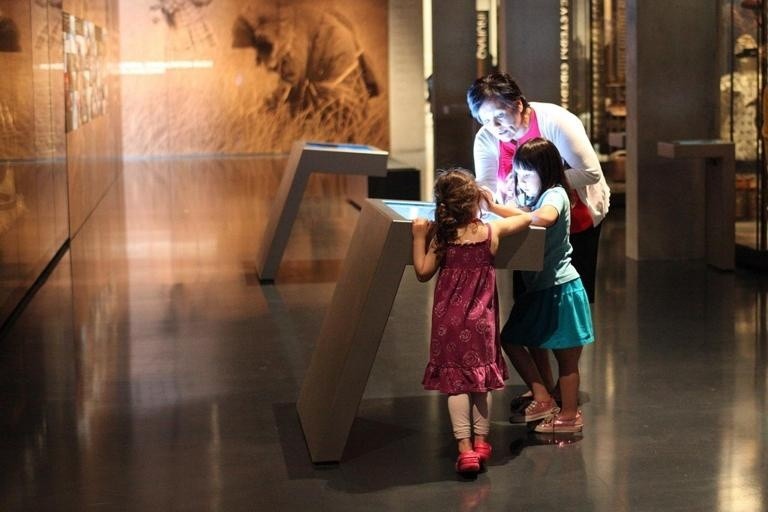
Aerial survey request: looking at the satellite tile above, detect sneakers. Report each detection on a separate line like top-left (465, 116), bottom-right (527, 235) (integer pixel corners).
top-left (454, 451), bottom-right (480, 472)
top-left (509, 386), bottom-right (583, 434)
top-left (474, 440), bottom-right (492, 459)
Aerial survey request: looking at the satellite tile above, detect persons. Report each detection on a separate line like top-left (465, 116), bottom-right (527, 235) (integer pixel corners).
top-left (411, 168), bottom-right (531, 475)
top-left (505, 139), bottom-right (595, 431)
top-left (466, 73), bottom-right (610, 411)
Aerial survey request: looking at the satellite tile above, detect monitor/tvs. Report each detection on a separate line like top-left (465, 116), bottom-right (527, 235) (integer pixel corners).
top-left (305, 142), bottom-right (377, 152)
top-left (381, 199), bottom-right (506, 221)
